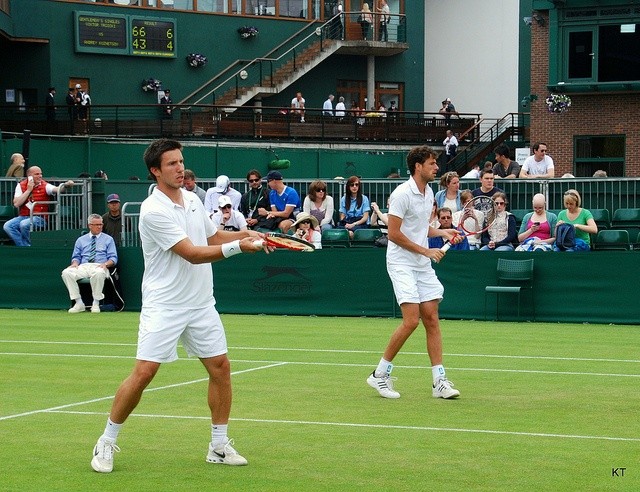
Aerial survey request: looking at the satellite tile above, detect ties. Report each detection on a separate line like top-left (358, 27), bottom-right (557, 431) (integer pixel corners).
top-left (88, 236), bottom-right (96, 263)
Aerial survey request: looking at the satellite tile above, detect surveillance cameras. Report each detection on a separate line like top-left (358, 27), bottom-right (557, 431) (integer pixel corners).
top-left (523, 16), bottom-right (540, 28)
top-left (522, 95), bottom-right (533, 107)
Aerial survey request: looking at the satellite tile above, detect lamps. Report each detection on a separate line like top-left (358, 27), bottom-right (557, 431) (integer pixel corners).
top-left (522, 95), bottom-right (537, 107)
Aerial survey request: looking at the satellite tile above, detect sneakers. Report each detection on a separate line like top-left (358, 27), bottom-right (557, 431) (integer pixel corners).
top-left (366, 370), bottom-right (401, 399)
top-left (91, 435), bottom-right (121, 473)
top-left (205, 438), bottom-right (247, 466)
top-left (431, 377), bottom-right (460, 399)
top-left (91, 304), bottom-right (100, 313)
top-left (68, 303), bottom-right (86, 313)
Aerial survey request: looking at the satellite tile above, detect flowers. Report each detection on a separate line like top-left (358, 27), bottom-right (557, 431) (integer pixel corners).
top-left (546, 94), bottom-right (572, 113)
top-left (186, 53), bottom-right (208, 67)
top-left (141, 78), bottom-right (161, 92)
top-left (236, 26), bottom-right (258, 39)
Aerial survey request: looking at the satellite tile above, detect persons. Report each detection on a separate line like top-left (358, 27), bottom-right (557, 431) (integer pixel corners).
top-left (101, 193), bottom-right (132, 246)
top-left (428, 206), bottom-right (470, 250)
top-left (89, 139), bottom-right (248, 472)
top-left (519, 142), bottom-right (554, 178)
top-left (258, 170), bottom-right (301, 234)
top-left (160, 89), bottom-right (173, 139)
top-left (366, 145), bottom-right (460, 399)
top-left (553, 189), bottom-right (598, 251)
top-left (5, 153), bottom-right (25, 177)
top-left (350, 100), bottom-right (359, 141)
top-left (94, 169), bottom-right (109, 180)
top-left (365, 107), bottom-right (383, 141)
top-left (331, 0), bottom-right (345, 41)
top-left (373, 105), bottom-right (388, 142)
top-left (442, 130), bottom-right (459, 171)
top-left (238, 168), bottom-right (271, 230)
top-left (388, 101), bottom-right (401, 141)
top-left (338, 176), bottom-right (370, 239)
top-left (386, 173), bottom-right (401, 179)
top-left (46, 87), bottom-right (58, 134)
top-left (370, 197), bottom-right (391, 237)
top-left (182, 169), bottom-right (206, 206)
top-left (290, 212), bottom-right (323, 249)
top-left (429, 199), bottom-right (441, 229)
top-left (74, 84), bottom-right (89, 137)
top-left (376, 0), bottom-right (390, 42)
top-left (334, 96), bottom-right (349, 141)
top-left (514, 193), bottom-right (558, 251)
top-left (446, 98), bottom-right (456, 112)
top-left (461, 163), bottom-right (481, 178)
top-left (561, 172), bottom-right (575, 178)
top-left (452, 189), bottom-right (484, 251)
top-left (479, 191), bottom-right (516, 251)
top-left (78, 173), bottom-right (92, 179)
top-left (484, 161), bottom-right (493, 170)
top-left (470, 168), bottom-right (507, 245)
top-left (357, 108), bottom-right (369, 141)
top-left (376, 101), bottom-right (382, 106)
top-left (322, 94), bottom-right (334, 119)
top-left (148, 173), bottom-right (154, 180)
top-left (434, 171), bottom-right (465, 212)
top-left (203, 174), bottom-right (242, 214)
top-left (2, 165), bottom-right (74, 246)
top-left (60, 213), bottom-right (119, 313)
top-left (492, 144), bottom-right (521, 179)
top-left (303, 179), bottom-right (334, 234)
top-left (221, 239), bottom-right (244, 259)
top-left (211, 195), bottom-right (248, 232)
top-left (439, 98), bottom-right (458, 127)
top-left (127, 175), bottom-right (141, 181)
top-left (593, 170), bottom-right (607, 178)
top-left (289, 92), bottom-right (306, 123)
top-left (66, 87), bottom-right (77, 135)
top-left (360, 3), bottom-right (372, 41)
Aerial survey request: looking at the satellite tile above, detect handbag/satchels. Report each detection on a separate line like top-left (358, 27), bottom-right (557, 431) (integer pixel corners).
top-left (357, 15), bottom-right (362, 23)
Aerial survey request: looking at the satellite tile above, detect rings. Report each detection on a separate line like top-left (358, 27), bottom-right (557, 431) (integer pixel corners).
top-left (534, 228), bottom-right (535, 230)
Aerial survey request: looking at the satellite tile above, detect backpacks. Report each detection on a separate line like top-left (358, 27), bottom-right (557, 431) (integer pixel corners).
top-left (80, 92), bottom-right (91, 106)
top-left (555, 223), bottom-right (576, 250)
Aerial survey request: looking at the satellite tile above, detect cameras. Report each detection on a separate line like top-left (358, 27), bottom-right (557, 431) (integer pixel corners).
top-left (299, 229), bottom-right (306, 235)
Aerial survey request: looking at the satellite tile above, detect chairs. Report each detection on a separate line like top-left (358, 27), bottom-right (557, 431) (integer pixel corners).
top-left (333, 210), bottom-right (337, 226)
top-left (586, 209), bottom-right (611, 244)
top-left (288, 229), bottom-right (295, 235)
top-left (371, 209), bottom-right (388, 214)
top-left (592, 230), bottom-right (633, 251)
top-left (0, 206), bottom-right (15, 246)
top-left (50, 206), bottom-right (80, 230)
top-left (485, 258), bottom-right (535, 322)
top-left (257, 227), bottom-right (282, 233)
top-left (321, 229), bottom-right (350, 248)
top-left (70, 278), bottom-right (104, 311)
top-left (509, 209), bottom-right (564, 237)
top-left (349, 229), bottom-right (383, 248)
top-left (612, 208), bottom-right (640, 245)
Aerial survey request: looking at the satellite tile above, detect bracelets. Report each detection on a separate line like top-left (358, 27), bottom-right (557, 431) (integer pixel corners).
top-left (220, 222), bottom-right (225, 226)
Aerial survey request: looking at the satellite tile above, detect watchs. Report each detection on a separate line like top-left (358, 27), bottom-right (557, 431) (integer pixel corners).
top-left (266, 211), bottom-right (270, 215)
top-left (105, 263), bottom-right (110, 268)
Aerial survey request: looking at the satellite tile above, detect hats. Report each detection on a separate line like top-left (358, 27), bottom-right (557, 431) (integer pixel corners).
top-left (164, 89), bottom-right (170, 93)
top-left (390, 100), bottom-right (396, 104)
top-left (107, 193), bottom-right (121, 202)
top-left (75, 83), bottom-right (81, 88)
top-left (290, 212), bottom-right (319, 233)
top-left (48, 87), bottom-right (55, 90)
top-left (217, 195), bottom-right (232, 208)
top-left (69, 87), bottom-right (74, 90)
top-left (215, 174), bottom-right (231, 193)
top-left (262, 170), bottom-right (283, 180)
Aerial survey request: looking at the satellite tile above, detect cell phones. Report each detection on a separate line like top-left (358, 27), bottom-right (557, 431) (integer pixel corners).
top-left (465, 208), bottom-right (471, 214)
top-left (28, 176), bottom-right (33, 182)
top-left (534, 222), bottom-right (540, 228)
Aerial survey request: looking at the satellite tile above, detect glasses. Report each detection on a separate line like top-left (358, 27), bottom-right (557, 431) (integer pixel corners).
top-left (89, 223), bottom-right (104, 226)
top-left (350, 183), bottom-right (359, 187)
top-left (221, 204), bottom-right (231, 209)
top-left (446, 172), bottom-right (457, 183)
top-left (316, 187), bottom-right (325, 192)
top-left (493, 201), bottom-right (504, 207)
top-left (440, 216), bottom-right (451, 219)
top-left (247, 178), bottom-right (260, 183)
top-left (537, 150), bottom-right (548, 153)
top-left (267, 179), bottom-right (275, 183)
top-left (300, 219), bottom-right (310, 224)
top-left (564, 191), bottom-right (576, 197)
top-left (532, 204), bottom-right (544, 211)
